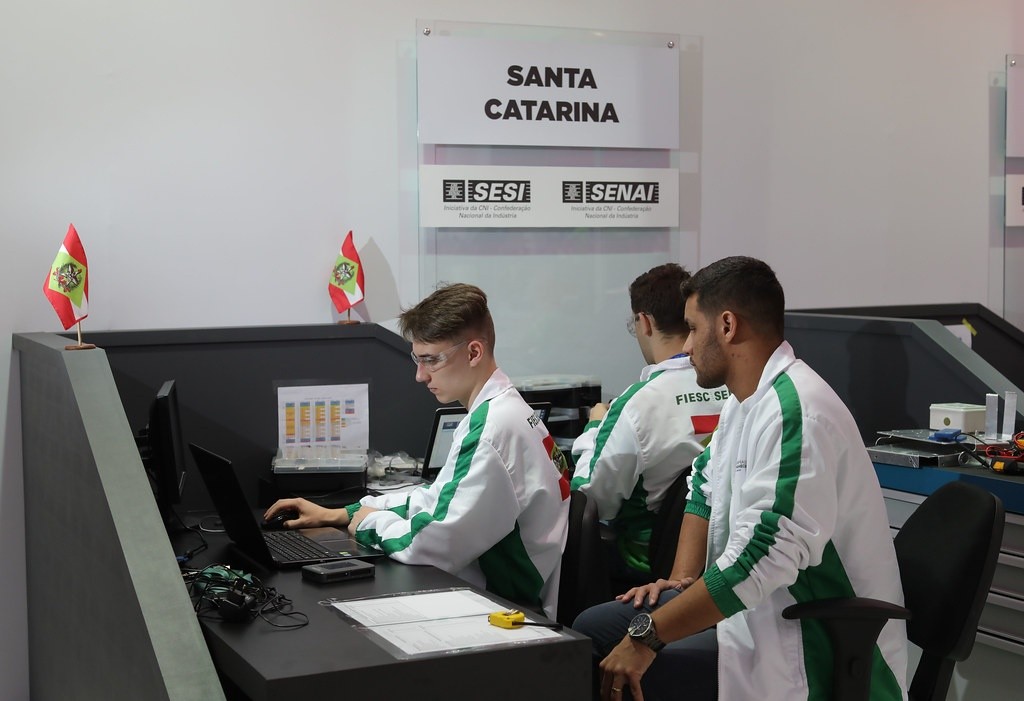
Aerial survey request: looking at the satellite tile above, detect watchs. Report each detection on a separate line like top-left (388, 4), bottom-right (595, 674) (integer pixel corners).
top-left (628, 613), bottom-right (665, 653)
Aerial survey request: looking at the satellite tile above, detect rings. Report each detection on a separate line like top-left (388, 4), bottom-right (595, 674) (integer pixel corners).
top-left (611, 687), bottom-right (622, 692)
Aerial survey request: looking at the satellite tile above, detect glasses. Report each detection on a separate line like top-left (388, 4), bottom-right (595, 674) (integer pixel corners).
top-left (410, 340), bottom-right (467, 372)
top-left (626, 312), bottom-right (644, 338)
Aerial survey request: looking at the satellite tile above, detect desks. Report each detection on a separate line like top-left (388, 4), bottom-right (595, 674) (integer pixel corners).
top-left (162, 491), bottom-right (596, 701)
top-left (861, 444), bottom-right (1024, 653)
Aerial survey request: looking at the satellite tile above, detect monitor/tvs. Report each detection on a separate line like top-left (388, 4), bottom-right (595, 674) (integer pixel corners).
top-left (149, 380), bottom-right (190, 504)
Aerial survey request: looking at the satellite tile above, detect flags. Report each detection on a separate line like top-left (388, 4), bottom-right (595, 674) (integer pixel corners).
top-left (329, 231), bottom-right (364, 313)
top-left (43, 223), bottom-right (88, 330)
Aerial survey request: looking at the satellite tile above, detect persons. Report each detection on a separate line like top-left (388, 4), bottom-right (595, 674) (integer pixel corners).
top-left (573, 256), bottom-right (908, 701)
top-left (568, 262), bottom-right (733, 590)
top-left (263, 283), bottom-right (570, 624)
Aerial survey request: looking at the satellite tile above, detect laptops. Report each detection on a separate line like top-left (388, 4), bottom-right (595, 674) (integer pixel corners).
top-left (188, 443), bottom-right (386, 571)
top-left (421, 401), bottom-right (552, 485)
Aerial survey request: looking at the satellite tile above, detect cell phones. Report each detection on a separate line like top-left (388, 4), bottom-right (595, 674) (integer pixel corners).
top-left (302, 559), bottom-right (376, 584)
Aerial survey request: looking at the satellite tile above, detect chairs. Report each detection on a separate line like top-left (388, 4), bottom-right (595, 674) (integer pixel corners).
top-left (782, 481), bottom-right (1006, 701)
top-left (606, 463), bottom-right (696, 601)
top-left (558, 491), bottom-right (606, 626)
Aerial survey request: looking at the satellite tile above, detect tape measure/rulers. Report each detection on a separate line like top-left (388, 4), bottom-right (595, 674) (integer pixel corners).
top-left (487, 608), bottom-right (564, 631)
